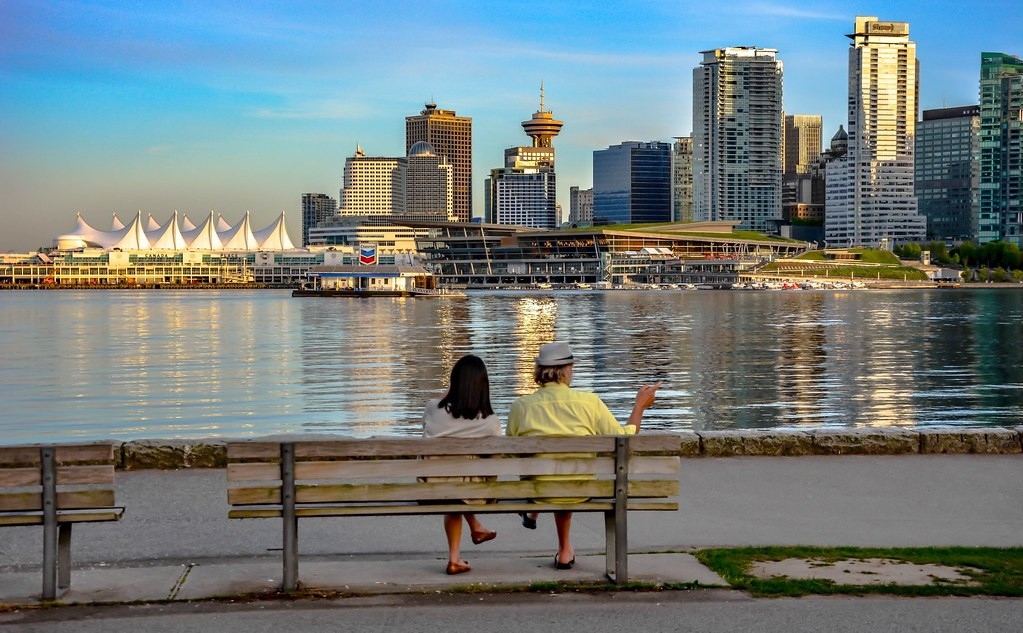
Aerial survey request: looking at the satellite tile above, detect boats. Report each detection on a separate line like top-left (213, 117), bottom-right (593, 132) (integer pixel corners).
top-left (291, 265), bottom-right (466, 298)
top-left (643, 282), bottom-right (697, 291)
top-left (577, 284), bottom-right (592, 291)
top-left (717, 278), bottom-right (866, 290)
top-left (533, 280), bottom-right (552, 289)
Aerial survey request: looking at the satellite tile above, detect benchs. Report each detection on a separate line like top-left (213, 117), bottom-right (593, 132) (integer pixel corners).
top-left (0, 442), bottom-right (125, 600)
top-left (227, 435), bottom-right (682, 593)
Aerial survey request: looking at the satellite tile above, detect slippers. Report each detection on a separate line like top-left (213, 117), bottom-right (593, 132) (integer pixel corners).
top-left (555, 551), bottom-right (575, 569)
top-left (517, 512), bottom-right (537, 529)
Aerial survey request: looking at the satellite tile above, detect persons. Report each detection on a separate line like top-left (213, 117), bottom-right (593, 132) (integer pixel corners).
top-left (501, 342), bottom-right (663, 569)
top-left (416, 355), bottom-right (501, 575)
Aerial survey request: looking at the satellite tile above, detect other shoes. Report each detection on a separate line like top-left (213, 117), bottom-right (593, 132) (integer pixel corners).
top-left (446, 561), bottom-right (470, 574)
top-left (471, 530), bottom-right (496, 544)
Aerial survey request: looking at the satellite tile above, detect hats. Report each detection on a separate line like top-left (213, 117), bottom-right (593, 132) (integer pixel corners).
top-left (535, 343), bottom-right (583, 366)
